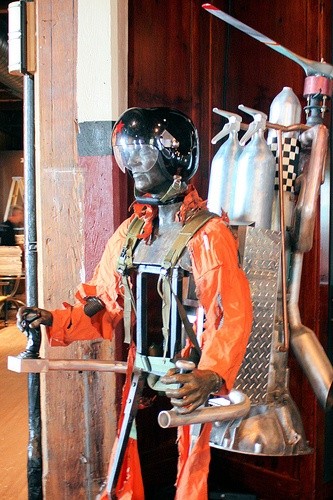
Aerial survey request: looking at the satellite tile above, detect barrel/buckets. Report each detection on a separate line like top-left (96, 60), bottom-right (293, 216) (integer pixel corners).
top-left (207, 444), bottom-right (317, 500)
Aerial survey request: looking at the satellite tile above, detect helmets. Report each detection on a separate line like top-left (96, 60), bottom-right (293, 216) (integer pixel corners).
top-left (110, 106), bottom-right (201, 195)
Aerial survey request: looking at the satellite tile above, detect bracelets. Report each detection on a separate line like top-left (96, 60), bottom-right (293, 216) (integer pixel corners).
top-left (213, 372), bottom-right (222, 393)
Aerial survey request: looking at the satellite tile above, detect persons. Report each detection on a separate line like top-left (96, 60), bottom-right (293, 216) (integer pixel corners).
top-left (17, 106), bottom-right (253, 500)
